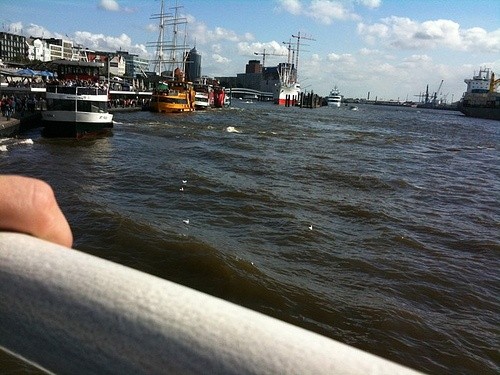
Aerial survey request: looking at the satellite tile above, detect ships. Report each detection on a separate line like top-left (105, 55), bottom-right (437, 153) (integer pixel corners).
top-left (274, 61), bottom-right (302, 105)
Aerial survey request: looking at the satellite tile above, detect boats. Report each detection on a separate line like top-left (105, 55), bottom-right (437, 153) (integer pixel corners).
top-left (460, 69), bottom-right (500, 119)
top-left (194, 78), bottom-right (230, 109)
top-left (326, 85), bottom-right (342, 108)
top-left (152, 78), bottom-right (196, 113)
top-left (39, 81), bottom-right (114, 140)
top-left (344, 80), bottom-right (460, 111)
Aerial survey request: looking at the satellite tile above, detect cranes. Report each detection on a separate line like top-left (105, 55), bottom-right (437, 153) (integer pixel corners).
top-left (255, 32), bottom-right (317, 73)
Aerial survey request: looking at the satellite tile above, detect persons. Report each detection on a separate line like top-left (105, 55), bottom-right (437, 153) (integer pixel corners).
top-left (1, 175), bottom-right (74, 250)
top-left (0, 76), bottom-right (154, 126)
